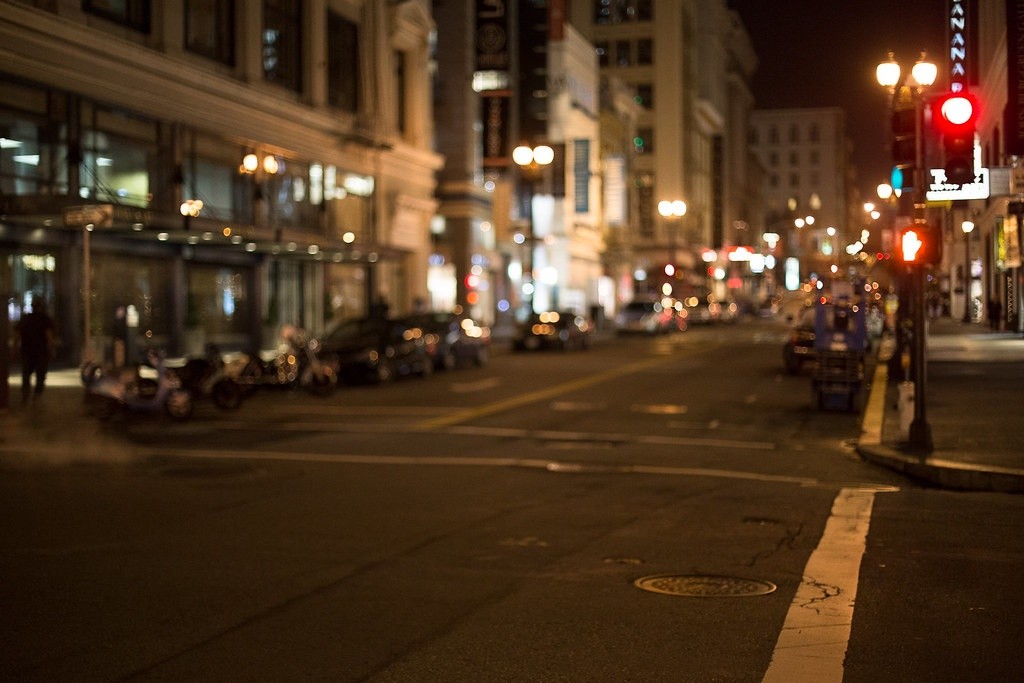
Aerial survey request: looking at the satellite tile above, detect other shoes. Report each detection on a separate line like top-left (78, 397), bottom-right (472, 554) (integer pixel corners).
top-left (34, 389), bottom-right (48, 398)
top-left (20, 389), bottom-right (32, 398)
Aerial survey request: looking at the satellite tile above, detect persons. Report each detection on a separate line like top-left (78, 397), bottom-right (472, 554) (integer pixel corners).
top-left (15, 304), bottom-right (54, 402)
top-left (114, 305), bottom-right (128, 365)
top-left (376, 296), bottom-right (390, 318)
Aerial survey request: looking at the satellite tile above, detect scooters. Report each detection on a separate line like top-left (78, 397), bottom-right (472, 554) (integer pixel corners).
top-left (80, 329), bottom-right (334, 427)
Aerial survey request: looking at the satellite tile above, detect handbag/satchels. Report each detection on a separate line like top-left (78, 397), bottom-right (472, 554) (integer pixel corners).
top-left (46, 333), bottom-right (63, 363)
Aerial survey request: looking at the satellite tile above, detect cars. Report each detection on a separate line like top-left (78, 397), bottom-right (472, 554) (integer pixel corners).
top-left (782, 300), bottom-right (885, 372)
top-left (511, 311), bottom-right (594, 350)
top-left (685, 298), bottom-right (719, 324)
top-left (615, 300), bottom-right (678, 332)
top-left (310, 310), bottom-right (489, 388)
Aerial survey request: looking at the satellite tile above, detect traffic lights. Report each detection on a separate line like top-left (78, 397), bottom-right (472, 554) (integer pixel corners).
top-left (891, 104), bottom-right (923, 199)
top-left (900, 227), bottom-right (927, 268)
top-left (941, 96), bottom-right (978, 184)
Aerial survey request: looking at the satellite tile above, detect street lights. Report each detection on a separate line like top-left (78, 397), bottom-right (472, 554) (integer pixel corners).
top-left (876, 50), bottom-right (939, 458)
top-left (514, 147), bottom-right (553, 311)
